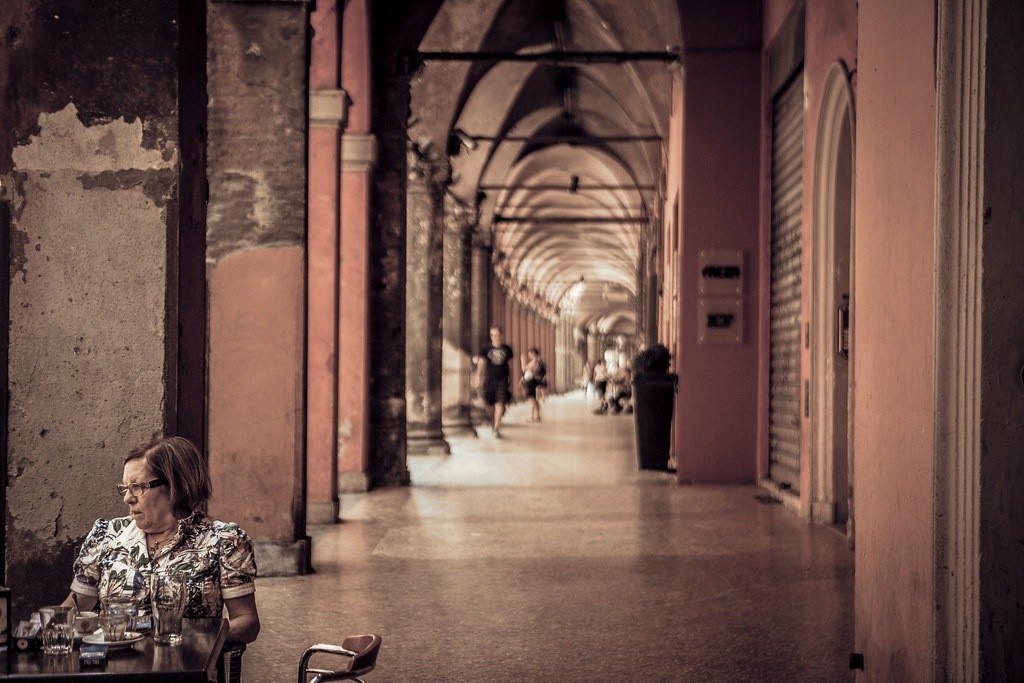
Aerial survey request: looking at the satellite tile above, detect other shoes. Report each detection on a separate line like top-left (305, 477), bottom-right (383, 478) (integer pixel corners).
top-left (493, 428), bottom-right (499, 438)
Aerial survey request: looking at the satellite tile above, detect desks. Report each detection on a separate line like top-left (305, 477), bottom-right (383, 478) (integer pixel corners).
top-left (0, 617), bottom-right (230, 683)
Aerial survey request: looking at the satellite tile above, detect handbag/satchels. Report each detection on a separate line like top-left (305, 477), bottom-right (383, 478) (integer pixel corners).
top-left (531, 358), bottom-right (546, 381)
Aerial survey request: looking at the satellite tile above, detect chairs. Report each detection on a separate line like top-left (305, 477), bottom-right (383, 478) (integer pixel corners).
top-left (297, 634), bottom-right (382, 683)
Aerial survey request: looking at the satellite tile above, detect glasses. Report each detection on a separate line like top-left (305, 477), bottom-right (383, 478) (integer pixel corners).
top-left (116, 479), bottom-right (164, 496)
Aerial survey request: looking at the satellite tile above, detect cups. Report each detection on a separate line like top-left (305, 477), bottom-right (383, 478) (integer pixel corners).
top-left (75, 612), bottom-right (98, 634)
top-left (100, 614), bottom-right (125, 640)
top-left (150, 573), bottom-right (185, 642)
top-left (39, 606), bottom-right (74, 654)
top-left (100, 597), bottom-right (139, 631)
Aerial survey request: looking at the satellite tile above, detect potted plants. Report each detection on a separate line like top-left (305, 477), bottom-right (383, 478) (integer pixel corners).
top-left (632, 342), bottom-right (671, 374)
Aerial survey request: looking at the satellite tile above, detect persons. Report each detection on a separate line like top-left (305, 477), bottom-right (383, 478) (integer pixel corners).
top-left (579, 357), bottom-right (631, 414)
top-left (54, 436), bottom-right (260, 652)
top-left (475, 326), bottom-right (513, 439)
top-left (516, 347), bottom-right (545, 424)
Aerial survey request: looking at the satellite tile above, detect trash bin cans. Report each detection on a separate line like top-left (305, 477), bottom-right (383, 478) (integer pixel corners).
top-left (630, 373), bottom-right (678, 470)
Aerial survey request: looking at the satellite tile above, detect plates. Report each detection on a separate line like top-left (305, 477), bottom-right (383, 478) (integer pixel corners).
top-left (81, 632), bottom-right (144, 650)
